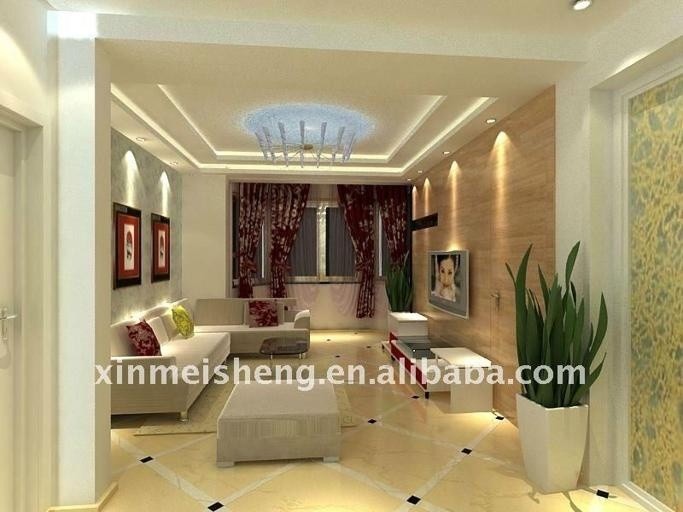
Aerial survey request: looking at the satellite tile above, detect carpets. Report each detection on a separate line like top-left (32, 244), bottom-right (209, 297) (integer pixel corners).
top-left (134, 358), bottom-right (359, 437)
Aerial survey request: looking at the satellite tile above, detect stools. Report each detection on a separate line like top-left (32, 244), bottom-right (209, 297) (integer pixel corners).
top-left (216, 379), bottom-right (340, 469)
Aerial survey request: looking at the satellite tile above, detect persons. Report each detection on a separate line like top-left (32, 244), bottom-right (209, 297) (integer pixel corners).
top-left (431, 254), bottom-right (461, 303)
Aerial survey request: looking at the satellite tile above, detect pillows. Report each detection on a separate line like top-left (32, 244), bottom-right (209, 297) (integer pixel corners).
top-left (171, 306), bottom-right (194, 339)
top-left (247, 299), bottom-right (278, 326)
top-left (126, 320), bottom-right (161, 356)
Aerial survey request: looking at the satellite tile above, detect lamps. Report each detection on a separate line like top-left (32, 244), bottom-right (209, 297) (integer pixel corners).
top-left (243, 106), bottom-right (376, 169)
top-left (572, 0), bottom-right (591, 14)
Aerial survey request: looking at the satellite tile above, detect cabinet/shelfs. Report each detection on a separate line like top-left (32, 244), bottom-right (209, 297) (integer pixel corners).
top-left (383, 310), bottom-right (493, 412)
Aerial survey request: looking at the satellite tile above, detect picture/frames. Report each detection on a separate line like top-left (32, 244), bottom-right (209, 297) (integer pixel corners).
top-left (113, 202), bottom-right (142, 290)
top-left (151, 213), bottom-right (170, 283)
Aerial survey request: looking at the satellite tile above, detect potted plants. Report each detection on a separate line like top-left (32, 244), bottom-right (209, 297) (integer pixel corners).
top-left (506, 241), bottom-right (609, 495)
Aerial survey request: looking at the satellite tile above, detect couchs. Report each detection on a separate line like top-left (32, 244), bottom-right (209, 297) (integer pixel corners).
top-left (194, 297), bottom-right (310, 358)
top-left (111, 298), bottom-right (231, 423)
